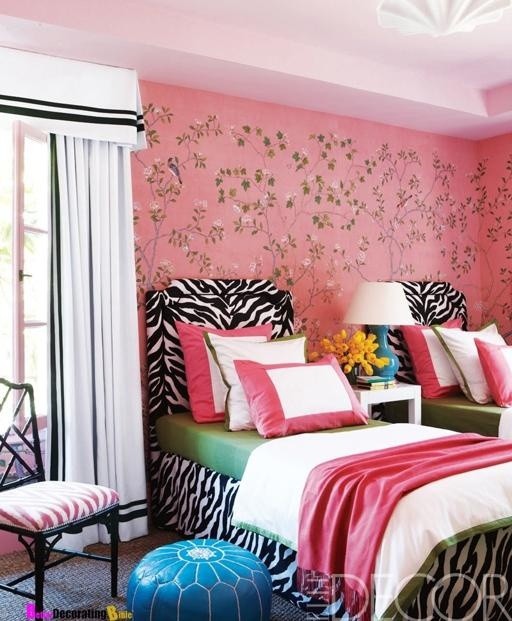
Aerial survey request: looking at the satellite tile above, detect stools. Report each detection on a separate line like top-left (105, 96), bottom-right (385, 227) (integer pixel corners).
top-left (126, 539), bottom-right (272, 620)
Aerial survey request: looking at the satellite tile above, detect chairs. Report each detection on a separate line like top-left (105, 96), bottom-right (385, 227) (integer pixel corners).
top-left (0, 378), bottom-right (120, 621)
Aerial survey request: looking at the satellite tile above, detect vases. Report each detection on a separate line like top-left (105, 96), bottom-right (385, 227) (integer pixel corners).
top-left (341, 362), bottom-right (363, 386)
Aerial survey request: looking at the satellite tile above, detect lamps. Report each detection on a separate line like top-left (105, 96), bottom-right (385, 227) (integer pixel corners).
top-left (342, 282), bottom-right (416, 380)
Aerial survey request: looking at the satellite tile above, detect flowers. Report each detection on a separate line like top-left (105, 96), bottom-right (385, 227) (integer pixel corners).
top-left (310, 330), bottom-right (389, 376)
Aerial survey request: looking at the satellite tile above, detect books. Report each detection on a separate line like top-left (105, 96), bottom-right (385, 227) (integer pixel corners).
top-left (356, 375), bottom-right (389, 383)
top-left (358, 384), bottom-right (395, 391)
top-left (357, 381), bottom-right (393, 386)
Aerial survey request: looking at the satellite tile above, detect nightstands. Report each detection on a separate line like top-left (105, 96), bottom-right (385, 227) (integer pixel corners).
top-left (358, 380), bottom-right (424, 428)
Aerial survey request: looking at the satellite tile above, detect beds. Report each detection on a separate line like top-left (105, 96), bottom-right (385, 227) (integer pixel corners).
top-left (388, 280), bottom-right (512, 440)
top-left (144, 279), bottom-right (511, 621)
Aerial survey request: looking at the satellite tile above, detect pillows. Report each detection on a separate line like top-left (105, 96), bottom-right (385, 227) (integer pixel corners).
top-left (233, 354), bottom-right (368, 437)
top-left (175, 321), bottom-right (272, 423)
top-left (401, 318), bottom-right (463, 399)
top-left (472, 338), bottom-right (512, 406)
top-left (203, 330), bottom-right (306, 432)
top-left (431, 319), bottom-right (508, 405)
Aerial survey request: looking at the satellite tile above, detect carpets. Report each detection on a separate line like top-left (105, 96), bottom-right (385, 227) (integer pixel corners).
top-left (0, 542), bottom-right (320, 621)
